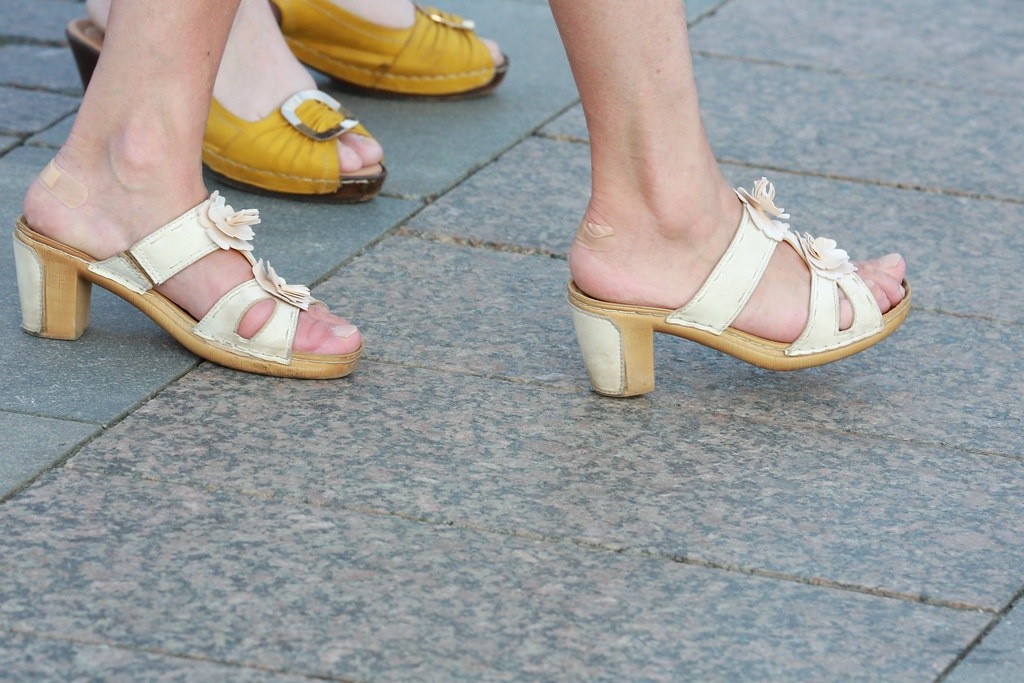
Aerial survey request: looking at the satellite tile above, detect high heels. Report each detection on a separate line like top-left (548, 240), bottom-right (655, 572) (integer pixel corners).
top-left (269, 0), bottom-right (509, 99)
top-left (66, 14), bottom-right (388, 203)
top-left (566, 176), bottom-right (911, 397)
top-left (12, 188), bottom-right (363, 379)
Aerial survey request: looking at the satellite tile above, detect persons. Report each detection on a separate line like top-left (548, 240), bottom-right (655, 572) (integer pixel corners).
top-left (64, 1), bottom-right (509, 201)
top-left (14, 1), bottom-right (912, 397)
